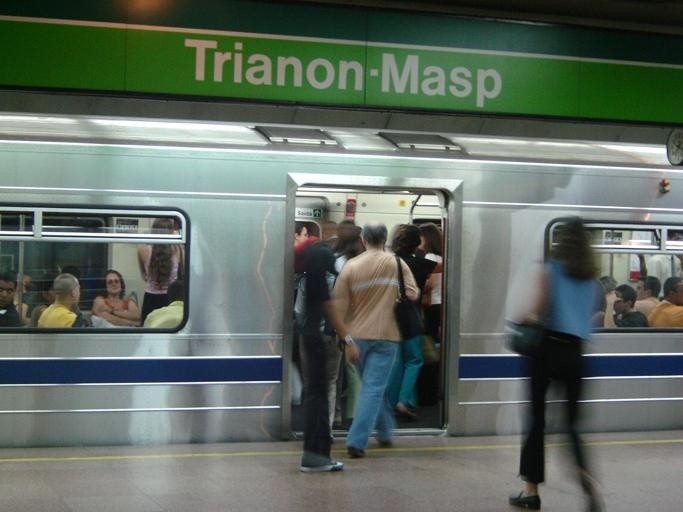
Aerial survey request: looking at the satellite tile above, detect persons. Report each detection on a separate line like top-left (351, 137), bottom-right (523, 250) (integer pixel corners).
top-left (0, 215), bottom-right (185, 331)
top-left (292, 221), bottom-right (442, 473)
top-left (589, 276), bottom-right (683, 327)
top-left (508, 216), bottom-right (618, 512)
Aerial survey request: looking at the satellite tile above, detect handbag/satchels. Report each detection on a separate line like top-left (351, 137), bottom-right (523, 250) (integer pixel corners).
top-left (507, 322), bottom-right (551, 361)
top-left (393, 255), bottom-right (426, 339)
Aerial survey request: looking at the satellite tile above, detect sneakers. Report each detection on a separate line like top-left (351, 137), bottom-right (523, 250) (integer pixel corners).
top-left (300, 460), bottom-right (344, 472)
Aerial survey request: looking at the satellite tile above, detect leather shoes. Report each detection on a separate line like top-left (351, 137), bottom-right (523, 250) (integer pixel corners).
top-left (509, 490), bottom-right (540, 509)
top-left (346, 445), bottom-right (366, 458)
